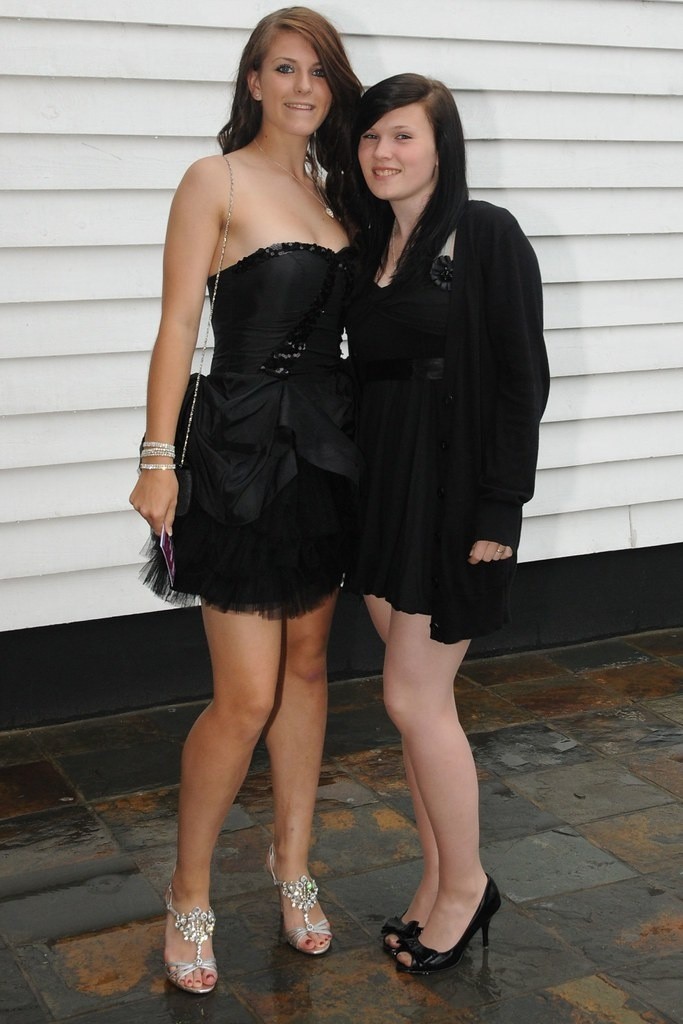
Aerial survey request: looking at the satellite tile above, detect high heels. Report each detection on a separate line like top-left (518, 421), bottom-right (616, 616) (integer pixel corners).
top-left (381, 908), bottom-right (424, 957)
top-left (393, 873), bottom-right (501, 975)
top-left (163, 866), bottom-right (218, 993)
top-left (266, 844), bottom-right (332, 956)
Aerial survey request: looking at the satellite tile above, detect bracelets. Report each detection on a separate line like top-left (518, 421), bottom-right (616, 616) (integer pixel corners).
top-left (139, 440), bottom-right (177, 470)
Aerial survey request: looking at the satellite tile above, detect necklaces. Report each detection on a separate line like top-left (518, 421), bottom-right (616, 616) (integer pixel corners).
top-left (254, 136), bottom-right (334, 219)
top-left (391, 216), bottom-right (401, 270)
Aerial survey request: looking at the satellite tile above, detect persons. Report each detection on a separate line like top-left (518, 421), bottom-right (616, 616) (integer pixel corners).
top-left (342, 73), bottom-right (551, 981)
top-left (129, 5), bottom-right (370, 994)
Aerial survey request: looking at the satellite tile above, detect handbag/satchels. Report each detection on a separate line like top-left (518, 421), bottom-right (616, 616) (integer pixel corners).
top-left (174, 469), bottom-right (192, 518)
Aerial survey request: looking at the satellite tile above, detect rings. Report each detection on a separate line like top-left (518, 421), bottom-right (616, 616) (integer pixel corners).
top-left (496, 550), bottom-right (504, 553)
